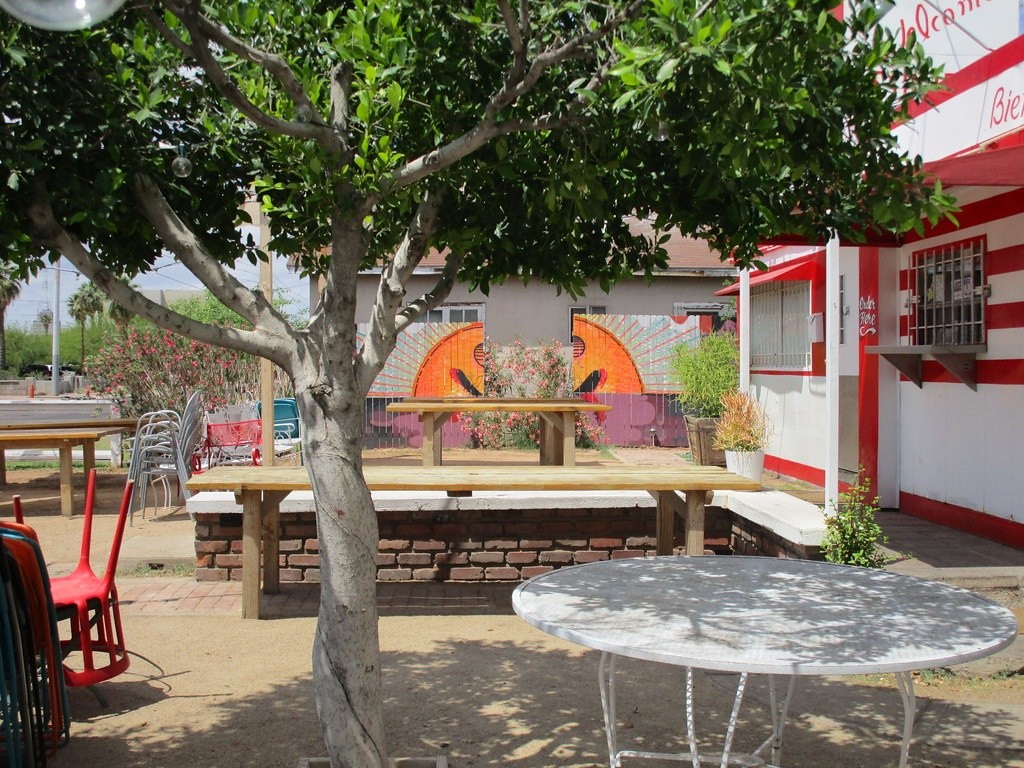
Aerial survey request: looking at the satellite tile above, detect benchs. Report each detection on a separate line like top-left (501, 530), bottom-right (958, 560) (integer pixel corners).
top-left (191, 419), bottom-right (263, 475)
top-left (386, 398), bottom-right (612, 467)
top-left (185, 465), bottom-right (762, 620)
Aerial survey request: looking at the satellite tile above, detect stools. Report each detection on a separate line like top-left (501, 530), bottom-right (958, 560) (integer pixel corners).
top-left (1, 468), bottom-right (134, 767)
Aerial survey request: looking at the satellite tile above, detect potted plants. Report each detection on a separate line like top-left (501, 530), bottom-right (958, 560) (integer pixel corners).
top-left (712, 388), bottom-right (766, 486)
top-left (667, 327), bottom-right (741, 468)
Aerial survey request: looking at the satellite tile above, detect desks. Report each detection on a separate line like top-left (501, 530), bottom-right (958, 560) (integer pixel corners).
top-left (0, 426), bottom-right (128, 515)
top-left (0, 417), bottom-right (180, 486)
top-left (510, 555), bottom-right (1019, 767)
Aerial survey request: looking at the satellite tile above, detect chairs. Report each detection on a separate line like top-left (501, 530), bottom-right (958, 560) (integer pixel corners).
top-left (127, 390), bottom-right (303, 527)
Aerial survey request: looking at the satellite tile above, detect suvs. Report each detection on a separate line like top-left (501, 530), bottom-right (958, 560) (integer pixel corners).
top-left (20, 365), bottom-right (64, 379)
